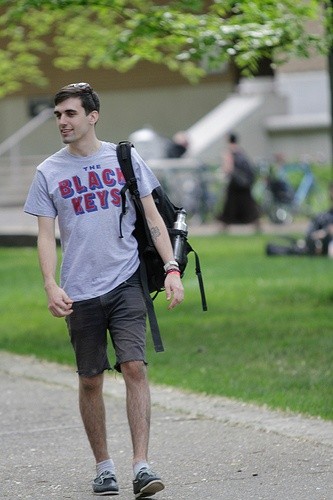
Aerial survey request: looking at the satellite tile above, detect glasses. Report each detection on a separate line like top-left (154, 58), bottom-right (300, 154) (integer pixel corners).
top-left (67, 82), bottom-right (98, 111)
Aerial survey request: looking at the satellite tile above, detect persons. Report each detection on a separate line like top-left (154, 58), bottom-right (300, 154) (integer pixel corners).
top-left (216, 133), bottom-right (264, 234)
top-left (23, 83), bottom-right (185, 500)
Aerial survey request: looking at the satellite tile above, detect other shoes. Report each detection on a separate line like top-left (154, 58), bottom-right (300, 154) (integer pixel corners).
top-left (132, 466), bottom-right (165, 499)
top-left (93, 470), bottom-right (120, 496)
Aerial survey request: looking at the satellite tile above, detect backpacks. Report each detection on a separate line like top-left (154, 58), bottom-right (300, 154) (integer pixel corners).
top-left (115, 140), bottom-right (208, 353)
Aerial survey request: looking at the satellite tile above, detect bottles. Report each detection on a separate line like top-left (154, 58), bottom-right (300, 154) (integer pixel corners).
top-left (172, 209), bottom-right (188, 262)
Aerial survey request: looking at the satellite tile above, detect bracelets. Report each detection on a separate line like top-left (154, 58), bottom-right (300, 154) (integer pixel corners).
top-left (164, 261), bottom-right (180, 275)
